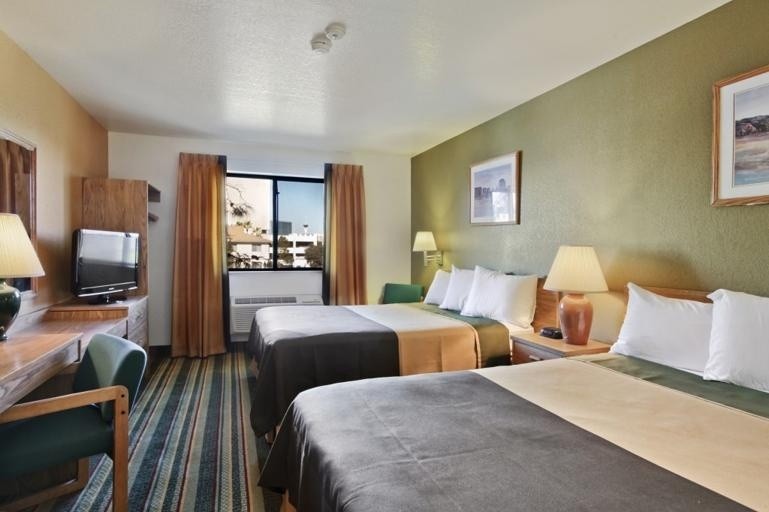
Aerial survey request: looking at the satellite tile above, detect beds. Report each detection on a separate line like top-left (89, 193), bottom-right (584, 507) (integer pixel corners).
top-left (244, 272), bottom-right (561, 447)
top-left (254, 288), bottom-right (768, 512)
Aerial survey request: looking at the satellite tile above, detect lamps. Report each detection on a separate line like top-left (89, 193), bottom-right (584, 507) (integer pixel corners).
top-left (542, 244), bottom-right (609, 345)
top-left (0, 209), bottom-right (46, 338)
top-left (412, 230), bottom-right (443, 268)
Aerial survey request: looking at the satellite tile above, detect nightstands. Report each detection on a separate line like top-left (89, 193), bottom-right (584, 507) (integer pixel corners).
top-left (510, 332), bottom-right (611, 366)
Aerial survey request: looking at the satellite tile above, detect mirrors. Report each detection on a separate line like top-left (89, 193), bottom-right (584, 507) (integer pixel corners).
top-left (0, 125), bottom-right (38, 304)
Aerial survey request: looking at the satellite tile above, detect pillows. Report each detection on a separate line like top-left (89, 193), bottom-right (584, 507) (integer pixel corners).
top-left (422, 268), bottom-right (451, 305)
top-left (607, 280), bottom-right (714, 377)
top-left (702, 289), bottom-right (769, 394)
top-left (459, 264), bottom-right (540, 329)
top-left (437, 264), bottom-right (475, 311)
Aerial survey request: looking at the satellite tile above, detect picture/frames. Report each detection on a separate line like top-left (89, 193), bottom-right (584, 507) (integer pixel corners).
top-left (710, 66), bottom-right (769, 208)
top-left (467, 148), bottom-right (523, 226)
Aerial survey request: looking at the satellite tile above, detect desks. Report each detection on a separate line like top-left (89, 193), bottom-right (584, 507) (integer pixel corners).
top-left (1, 317), bottom-right (128, 415)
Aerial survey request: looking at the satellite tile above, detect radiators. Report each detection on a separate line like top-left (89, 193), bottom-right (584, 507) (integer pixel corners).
top-left (229, 295), bottom-right (324, 336)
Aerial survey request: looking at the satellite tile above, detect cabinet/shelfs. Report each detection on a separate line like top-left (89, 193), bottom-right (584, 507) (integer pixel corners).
top-left (48, 295), bottom-right (150, 380)
top-left (83, 176), bottom-right (161, 295)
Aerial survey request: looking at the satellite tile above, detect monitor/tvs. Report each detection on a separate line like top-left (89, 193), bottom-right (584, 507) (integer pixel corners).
top-left (71, 228), bottom-right (142, 305)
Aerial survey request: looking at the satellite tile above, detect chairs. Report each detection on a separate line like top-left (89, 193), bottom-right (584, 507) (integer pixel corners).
top-left (1, 334), bottom-right (150, 510)
top-left (383, 282), bottom-right (423, 304)
